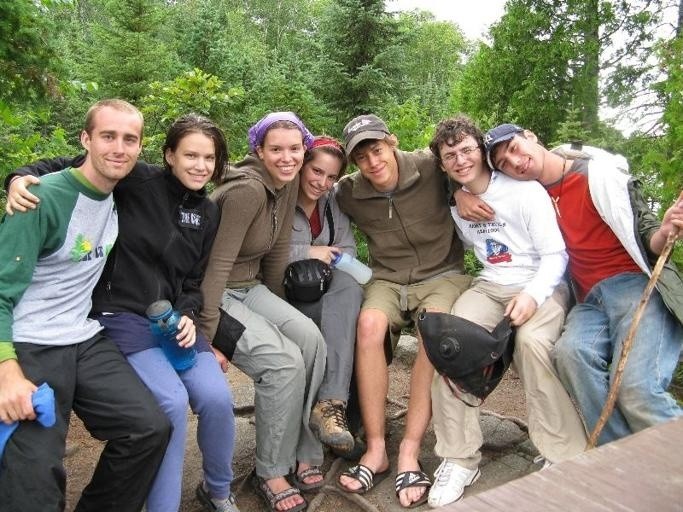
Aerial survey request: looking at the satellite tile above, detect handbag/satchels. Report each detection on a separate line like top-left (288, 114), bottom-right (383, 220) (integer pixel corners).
top-left (282, 255), bottom-right (336, 305)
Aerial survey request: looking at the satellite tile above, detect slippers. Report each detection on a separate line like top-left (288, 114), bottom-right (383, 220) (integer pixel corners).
top-left (394, 458), bottom-right (435, 510)
top-left (335, 462), bottom-right (393, 495)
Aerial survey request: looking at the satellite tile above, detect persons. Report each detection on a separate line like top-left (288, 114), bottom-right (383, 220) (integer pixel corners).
top-left (447, 122), bottom-right (683, 448)
top-left (201, 112), bottom-right (327, 512)
top-left (427, 117), bottom-right (589, 509)
top-left (283, 134), bottom-right (367, 460)
top-left (3, 113), bottom-right (241, 512)
top-left (333, 114), bottom-right (474, 508)
top-left (0, 97), bottom-right (175, 512)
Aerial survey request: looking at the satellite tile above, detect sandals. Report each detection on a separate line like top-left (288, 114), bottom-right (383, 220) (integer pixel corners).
top-left (249, 471), bottom-right (308, 512)
top-left (195, 476), bottom-right (242, 512)
top-left (286, 463), bottom-right (326, 491)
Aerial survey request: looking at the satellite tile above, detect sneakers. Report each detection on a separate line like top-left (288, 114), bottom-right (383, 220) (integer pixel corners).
top-left (426, 456), bottom-right (483, 510)
top-left (307, 397), bottom-right (369, 462)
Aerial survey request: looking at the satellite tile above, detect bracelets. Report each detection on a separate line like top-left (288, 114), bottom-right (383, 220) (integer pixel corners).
top-left (7, 176), bottom-right (21, 193)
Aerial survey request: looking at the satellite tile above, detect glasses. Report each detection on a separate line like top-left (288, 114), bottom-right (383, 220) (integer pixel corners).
top-left (437, 145), bottom-right (482, 163)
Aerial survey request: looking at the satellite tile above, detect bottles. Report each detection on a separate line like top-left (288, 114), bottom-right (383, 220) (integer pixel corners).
top-left (336, 250), bottom-right (373, 286)
top-left (146, 299), bottom-right (197, 371)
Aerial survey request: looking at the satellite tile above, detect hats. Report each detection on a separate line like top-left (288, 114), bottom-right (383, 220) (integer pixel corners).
top-left (341, 113), bottom-right (395, 157)
top-left (482, 123), bottom-right (526, 174)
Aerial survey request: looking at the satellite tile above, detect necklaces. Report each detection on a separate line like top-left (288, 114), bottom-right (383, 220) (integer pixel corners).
top-left (544, 155), bottom-right (566, 218)
